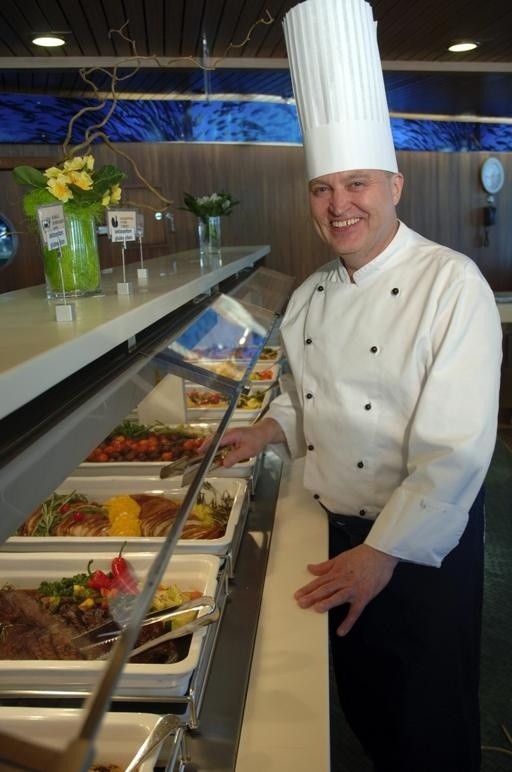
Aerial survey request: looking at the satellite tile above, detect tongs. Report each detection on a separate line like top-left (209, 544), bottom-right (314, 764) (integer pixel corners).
top-left (76, 594), bottom-right (221, 664)
top-left (158, 442), bottom-right (236, 489)
top-left (121, 714), bottom-right (185, 772)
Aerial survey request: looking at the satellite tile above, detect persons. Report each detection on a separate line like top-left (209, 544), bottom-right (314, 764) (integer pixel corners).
top-left (198, 170), bottom-right (504, 772)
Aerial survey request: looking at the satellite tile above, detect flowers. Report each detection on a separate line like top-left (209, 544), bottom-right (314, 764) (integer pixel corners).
top-left (14, 157), bottom-right (122, 289)
top-left (174, 189), bottom-right (242, 245)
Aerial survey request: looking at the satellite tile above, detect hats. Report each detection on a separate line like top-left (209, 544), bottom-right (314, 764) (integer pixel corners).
top-left (280, 0), bottom-right (399, 183)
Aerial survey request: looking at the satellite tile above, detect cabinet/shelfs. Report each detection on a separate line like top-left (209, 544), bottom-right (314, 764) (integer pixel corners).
top-left (0, 292), bottom-right (331, 772)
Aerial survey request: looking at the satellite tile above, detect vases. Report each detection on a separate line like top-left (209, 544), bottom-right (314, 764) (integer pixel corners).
top-left (197, 216), bottom-right (221, 253)
top-left (42, 216), bottom-right (101, 296)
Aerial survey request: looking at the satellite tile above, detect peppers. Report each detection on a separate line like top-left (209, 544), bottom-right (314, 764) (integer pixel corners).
top-left (111, 540), bottom-right (140, 597)
top-left (85, 559), bottom-right (112, 590)
